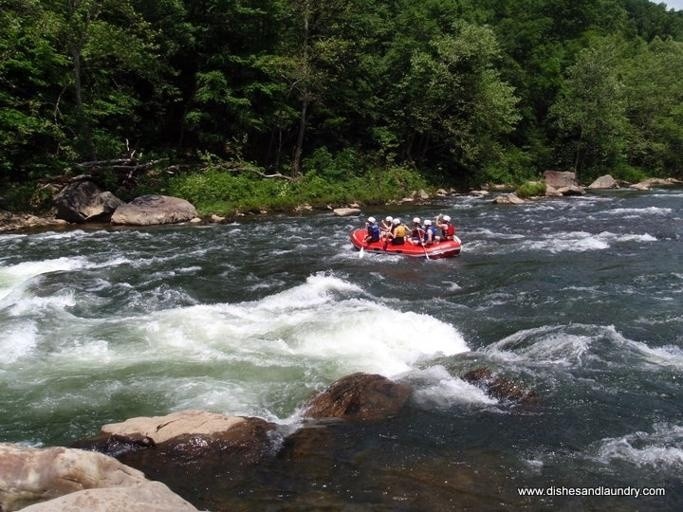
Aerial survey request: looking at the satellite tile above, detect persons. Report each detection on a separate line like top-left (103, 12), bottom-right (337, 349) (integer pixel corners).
top-left (362, 212), bottom-right (456, 247)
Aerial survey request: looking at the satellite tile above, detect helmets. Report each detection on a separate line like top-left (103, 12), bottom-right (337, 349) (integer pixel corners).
top-left (386, 216), bottom-right (401, 227)
top-left (368, 216), bottom-right (376, 224)
top-left (413, 217), bottom-right (421, 224)
top-left (424, 220), bottom-right (432, 226)
top-left (442, 215), bottom-right (451, 222)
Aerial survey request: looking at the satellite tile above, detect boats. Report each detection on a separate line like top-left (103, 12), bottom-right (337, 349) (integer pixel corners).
top-left (348, 228), bottom-right (462, 260)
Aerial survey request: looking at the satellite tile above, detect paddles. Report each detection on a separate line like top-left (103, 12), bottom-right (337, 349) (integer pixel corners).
top-left (358, 222), bottom-right (369, 257)
top-left (383, 223), bottom-right (393, 251)
